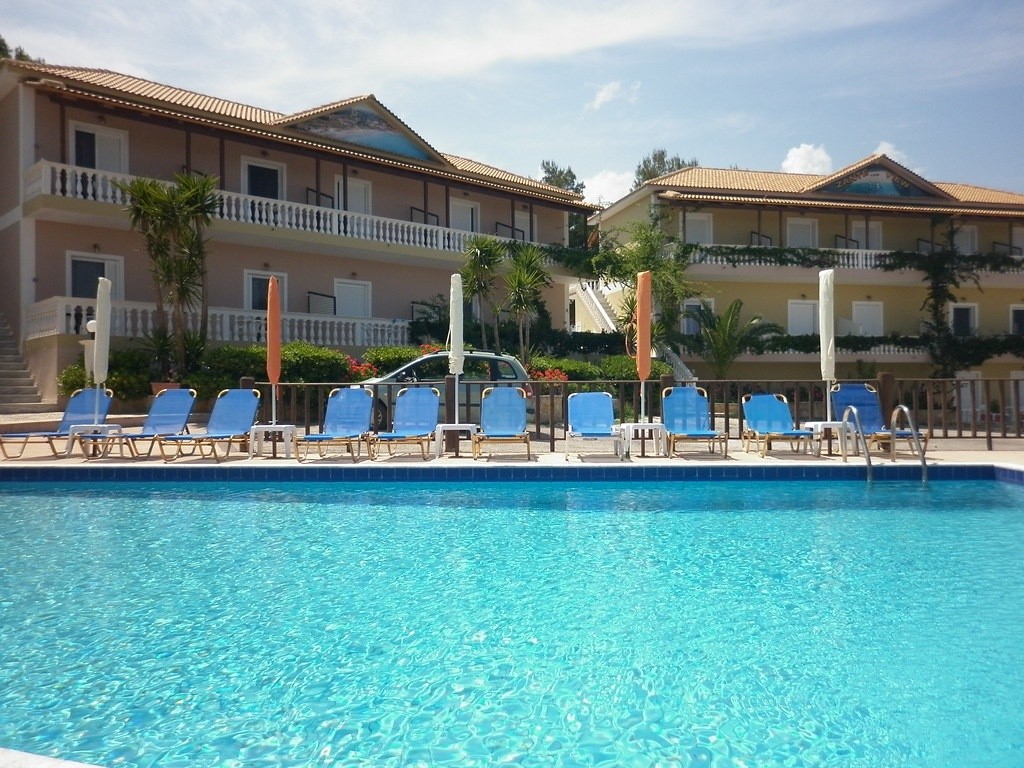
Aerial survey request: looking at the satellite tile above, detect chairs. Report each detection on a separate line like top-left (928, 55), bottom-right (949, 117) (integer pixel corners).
top-left (79, 386), bottom-right (197, 460)
top-left (564, 391), bottom-right (624, 461)
top-left (157, 388), bottom-right (261, 463)
top-left (829, 383), bottom-right (930, 460)
top-left (370, 386), bottom-right (441, 460)
top-left (471, 387), bottom-right (532, 461)
top-left (0, 387), bottom-right (114, 458)
top-left (294, 388), bottom-right (374, 463)
top-left (741, 392), bottom-right (823, 458)
top-left (662, 386), bottom-right (729, 460)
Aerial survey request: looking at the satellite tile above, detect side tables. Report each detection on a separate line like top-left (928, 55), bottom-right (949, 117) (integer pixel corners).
top-left (804, 420), bottom-right (860, 458)
top-left (434, 423), bottom-right (478, 459)
top-left (249, 423), bottom-right (299, 458)
top-left (620, 423), bottom-right (668, 459)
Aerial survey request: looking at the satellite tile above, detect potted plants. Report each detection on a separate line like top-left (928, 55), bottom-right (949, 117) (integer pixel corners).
top-left (133, 327), bottom-right (181, 395)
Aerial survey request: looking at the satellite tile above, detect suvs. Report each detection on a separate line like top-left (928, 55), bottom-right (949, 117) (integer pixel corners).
top-left (346, 348), bottom-right (536, 431)
